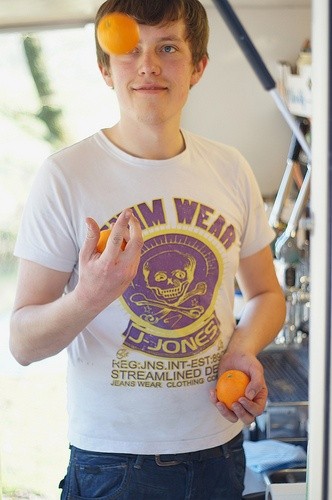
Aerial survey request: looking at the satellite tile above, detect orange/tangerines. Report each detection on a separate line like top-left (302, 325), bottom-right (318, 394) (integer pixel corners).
top-left (95, 228), bottom-right (127, 254)
top-left (98, 13), bottom-right (140, 56)
top-left (216, 369), bottom-right (250, 413)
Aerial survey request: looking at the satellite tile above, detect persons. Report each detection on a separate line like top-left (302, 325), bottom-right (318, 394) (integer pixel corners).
top-left (10, 0), bottom-right (287, 500)
